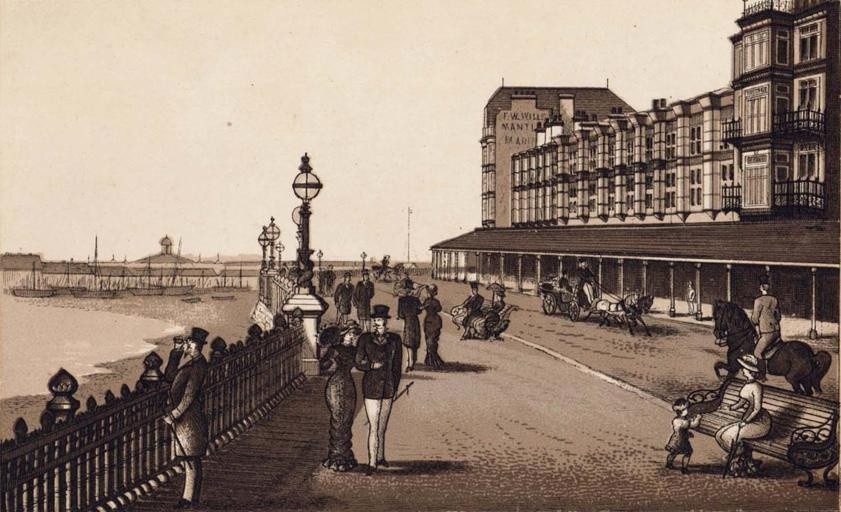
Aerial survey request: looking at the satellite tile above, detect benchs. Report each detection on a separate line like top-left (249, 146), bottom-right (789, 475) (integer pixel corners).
top-left (450, 296), bottom-right (520, 342)
top-left (392, 281), bottom-right (428, 299)
top-left (686, 377), bottom-right (840, 489)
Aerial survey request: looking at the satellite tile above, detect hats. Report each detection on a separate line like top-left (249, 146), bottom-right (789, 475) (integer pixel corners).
top-left (471, 283), bottom-right (477, 289)
top-left (671, 398), bottom-right (688, 410)
top-left (736, 355), bottom-right (760, 373)
top-left (338, 319), bottom-right (362, 335)
top-left (368, 304), bottom-right (390, 318)
top-left (186, 327), bottom-right (209, 346)
top-left (758, 274), bottom-right (769, 285)
top-left (403, 280), bottom-right (414, 291)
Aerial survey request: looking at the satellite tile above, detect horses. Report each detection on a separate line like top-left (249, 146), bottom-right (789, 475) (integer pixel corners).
top-left (589, 291), bottom-right (655, 337)
top-left (710, 296), bottom-right (832, 397)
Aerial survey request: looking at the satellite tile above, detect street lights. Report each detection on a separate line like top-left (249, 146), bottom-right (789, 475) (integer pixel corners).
top-left (291, 150), bottom-right (323, 289)
top-left (256, 217), bottom-right (284, 271)
top-left (360, 252), bottom-right (369, 271)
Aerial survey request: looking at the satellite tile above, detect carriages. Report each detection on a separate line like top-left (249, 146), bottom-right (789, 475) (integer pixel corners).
top-left (538, 272), bottom-right (654, 339)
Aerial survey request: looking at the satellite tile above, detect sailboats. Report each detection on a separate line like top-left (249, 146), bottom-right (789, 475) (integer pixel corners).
top-left (11, 233), bottom-right (251, 304)
